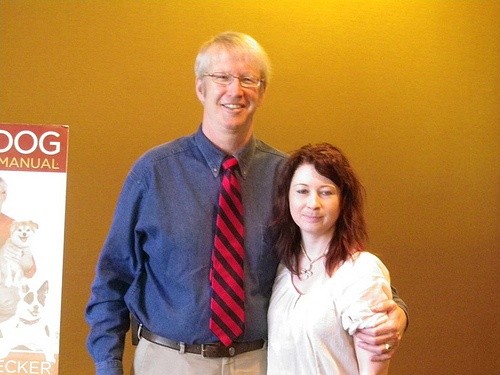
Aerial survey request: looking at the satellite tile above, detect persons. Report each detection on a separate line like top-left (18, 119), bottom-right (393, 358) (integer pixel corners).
top-left (267, 142), bottom-right (393, 375)
top-left (85, 32), bottom-right (409, 375)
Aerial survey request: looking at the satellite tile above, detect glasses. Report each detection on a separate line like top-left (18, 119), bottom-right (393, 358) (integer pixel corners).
top-left (202, 72), bottom-right (264, 87)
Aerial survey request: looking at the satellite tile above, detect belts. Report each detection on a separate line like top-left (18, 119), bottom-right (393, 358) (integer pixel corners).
top-left (140, 328), bottom-right (265, 358)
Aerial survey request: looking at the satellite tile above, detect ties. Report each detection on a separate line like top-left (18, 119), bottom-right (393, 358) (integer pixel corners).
top-left (209, 155), bottom-right (245, 346)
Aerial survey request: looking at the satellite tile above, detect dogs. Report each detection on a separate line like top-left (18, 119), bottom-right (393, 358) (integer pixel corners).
top-left (0, 220), bottom-right (39, 290)
top-left (0, 277), bottom-right (60, 362)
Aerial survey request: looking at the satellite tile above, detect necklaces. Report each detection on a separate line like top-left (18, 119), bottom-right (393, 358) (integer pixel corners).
top-left (299, 243), bottom-right (327, 279)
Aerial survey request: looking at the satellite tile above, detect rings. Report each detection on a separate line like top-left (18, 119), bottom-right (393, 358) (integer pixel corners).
top-left (385, 343), bottom-right (392, 351)
